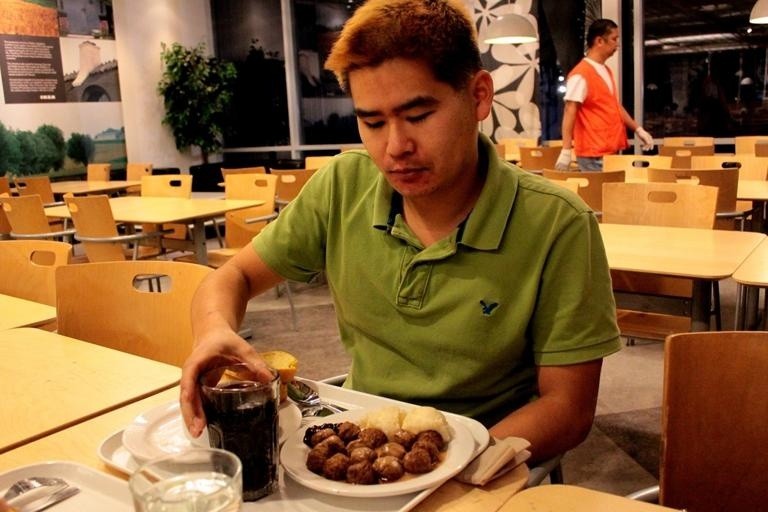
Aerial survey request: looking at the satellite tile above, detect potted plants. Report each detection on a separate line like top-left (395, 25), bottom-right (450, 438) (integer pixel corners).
top-left (152, 37), bottom-right (237, 193)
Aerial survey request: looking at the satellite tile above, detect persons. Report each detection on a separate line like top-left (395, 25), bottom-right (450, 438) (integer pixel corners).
top-left (180, 0), bottom-right (622, 471)
top-left (554, 18), bottom-right (655, 172)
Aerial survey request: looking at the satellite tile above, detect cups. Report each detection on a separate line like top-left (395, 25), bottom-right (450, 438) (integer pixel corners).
top-left (128, 447), bottom-right (244, 511)
top-left (196, 360), bottom-right (279, 500)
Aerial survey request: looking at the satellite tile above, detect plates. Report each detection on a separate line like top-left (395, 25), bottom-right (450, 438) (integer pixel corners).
top-left (0, 462), bottom-right (129, 512)
top-left (97, 372), bottom-right (490, 511)
top-left (279, 404), bottom-right (475, 497)
top-left (121, 393), bottom-right (302, 463)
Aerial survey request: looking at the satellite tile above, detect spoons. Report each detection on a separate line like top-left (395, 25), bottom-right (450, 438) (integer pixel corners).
top-left (286, 378), bottom-right (347, 412)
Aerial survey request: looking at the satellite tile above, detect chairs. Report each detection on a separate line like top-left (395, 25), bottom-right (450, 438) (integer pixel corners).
top-left (0, 118), bottom-right (768, 510)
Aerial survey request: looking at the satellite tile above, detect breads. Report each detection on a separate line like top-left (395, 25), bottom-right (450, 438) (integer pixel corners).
top-left (262, 351), bottom-right (297, 402)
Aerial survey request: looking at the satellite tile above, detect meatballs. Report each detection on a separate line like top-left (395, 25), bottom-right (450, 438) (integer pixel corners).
top-left (307, 422), bottom-right (444, 485)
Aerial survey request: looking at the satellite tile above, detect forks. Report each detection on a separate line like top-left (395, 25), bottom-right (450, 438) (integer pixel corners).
top-left (1, 473), bottom-right (64, 502)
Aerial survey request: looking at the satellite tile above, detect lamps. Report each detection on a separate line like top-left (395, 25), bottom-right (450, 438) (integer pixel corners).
top-left (748, 0), bottom-right (767, 24)
top-left (483, 1), bottom-right (538, 48)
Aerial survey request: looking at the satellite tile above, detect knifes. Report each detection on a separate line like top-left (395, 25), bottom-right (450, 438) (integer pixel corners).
top-left (19, 485), bottom-right (80, 510)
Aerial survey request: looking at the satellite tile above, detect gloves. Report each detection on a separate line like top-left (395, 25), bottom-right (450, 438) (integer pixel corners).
top-left (554, 149), bottom-right (571, 171)
top-left (635, 127), bottom-right (653, 151)
